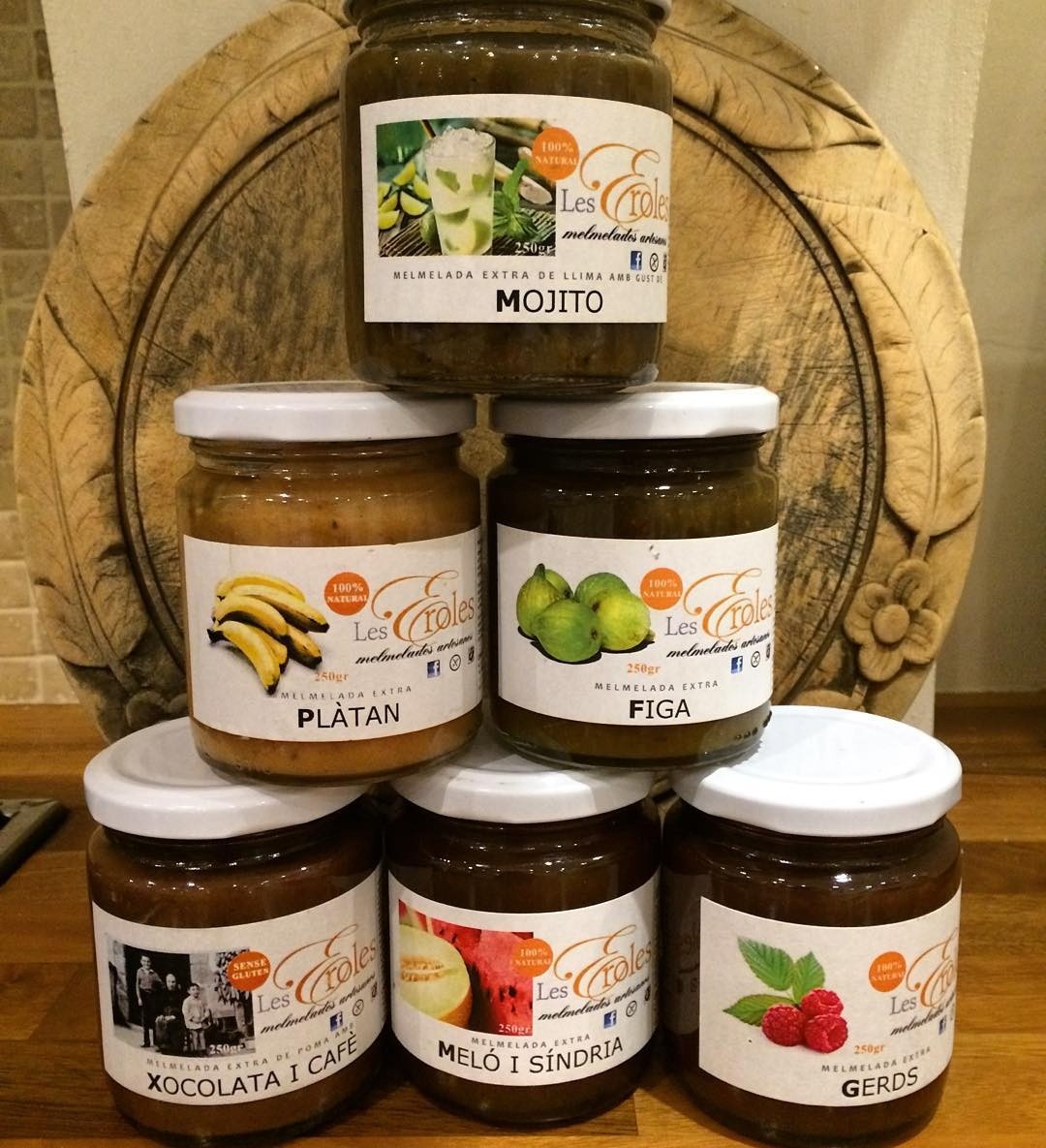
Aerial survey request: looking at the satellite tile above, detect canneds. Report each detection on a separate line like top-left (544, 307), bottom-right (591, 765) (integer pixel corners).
top-left (91, 4), bottom-right (963, 1148)
